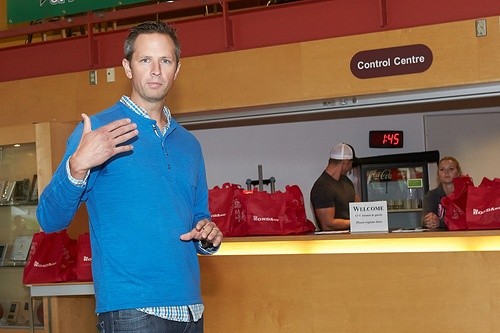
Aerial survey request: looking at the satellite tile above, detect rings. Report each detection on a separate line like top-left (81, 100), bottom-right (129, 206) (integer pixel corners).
top-left (214, 228), bottom-right (219, 233)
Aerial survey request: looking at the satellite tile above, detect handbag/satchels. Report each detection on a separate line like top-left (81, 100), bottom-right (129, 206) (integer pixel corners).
top-left (441, 174), bottom-right (474, 231)
top-left (208, 183), bottom-right (317, 237)
top-left (466, 177), bottom-right (500, 229)
top-left (23, 229), bottom-right (92, 285)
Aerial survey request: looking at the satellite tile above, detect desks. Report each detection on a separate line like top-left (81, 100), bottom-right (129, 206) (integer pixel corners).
top-left (29, 282), bottom-right (95, 333)
top-left (0, 123), bottom-right (76, 333)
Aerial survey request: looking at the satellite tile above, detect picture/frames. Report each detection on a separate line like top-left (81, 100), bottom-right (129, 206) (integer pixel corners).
top-left (5, 301), bottom-right (30, 322)
top-left (29, 175), bottom-right (38, 202)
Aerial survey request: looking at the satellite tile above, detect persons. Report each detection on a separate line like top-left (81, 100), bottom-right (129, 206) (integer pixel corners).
top-left (421, 156), bottom-right (462, 230)
top-left (36, 21), bottom-right (224, 333)
top-left (310, 142), bottom-right (360, 231)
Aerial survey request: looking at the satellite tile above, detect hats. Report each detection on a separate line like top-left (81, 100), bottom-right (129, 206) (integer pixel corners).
top-left (329, 143), bottom-right (362, 162)
